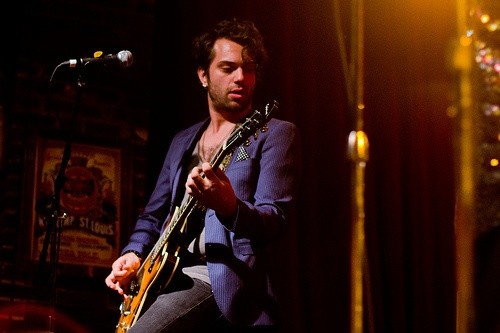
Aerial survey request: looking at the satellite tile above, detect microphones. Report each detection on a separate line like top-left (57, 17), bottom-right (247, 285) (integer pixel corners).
top-left (61, 50), bottom-right (133, 68)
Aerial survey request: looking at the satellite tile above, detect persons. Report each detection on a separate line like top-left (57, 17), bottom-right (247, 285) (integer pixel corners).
top-left (105, 21), bottom-right (303, 333)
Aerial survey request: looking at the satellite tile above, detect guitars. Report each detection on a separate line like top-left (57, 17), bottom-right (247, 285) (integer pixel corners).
top-left (113, 99), bottom-right (279, 333)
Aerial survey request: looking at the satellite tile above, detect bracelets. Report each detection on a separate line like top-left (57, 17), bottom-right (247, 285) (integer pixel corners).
top-left (122, 250), bottom-right (140, 257)
